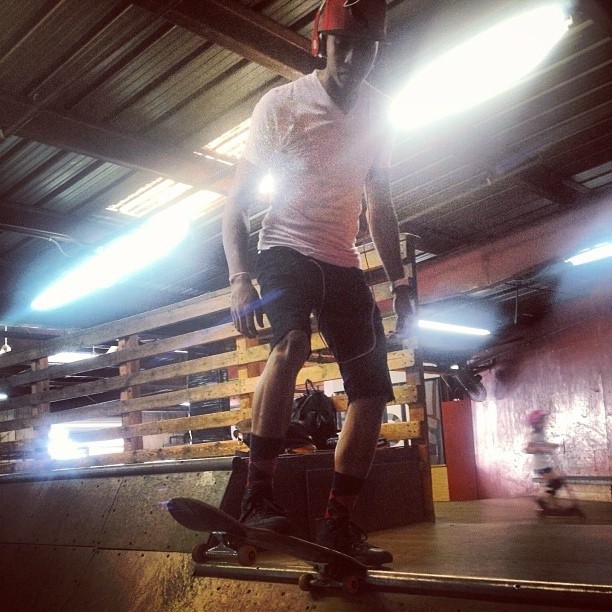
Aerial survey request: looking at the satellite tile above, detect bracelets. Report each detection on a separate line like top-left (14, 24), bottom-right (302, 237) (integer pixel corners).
top-left (229, 272), bottom-right (250, 284)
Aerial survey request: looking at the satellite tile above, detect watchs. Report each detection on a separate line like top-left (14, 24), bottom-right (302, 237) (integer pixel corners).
top-left (390, 276), bottom-right (416, 295)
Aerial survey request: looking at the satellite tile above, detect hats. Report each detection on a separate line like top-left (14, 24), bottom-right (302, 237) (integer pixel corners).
top-left (527, 411), bottom-right (548, 425)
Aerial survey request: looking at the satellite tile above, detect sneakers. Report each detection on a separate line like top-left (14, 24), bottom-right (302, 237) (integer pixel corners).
top-left (238, 485), bottom-right (291, 532)
top-left (315, 518), bottom-right (393, 565)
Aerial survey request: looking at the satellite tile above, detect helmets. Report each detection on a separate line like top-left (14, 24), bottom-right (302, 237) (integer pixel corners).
top-left (312, 0), bottom-right (389, 66)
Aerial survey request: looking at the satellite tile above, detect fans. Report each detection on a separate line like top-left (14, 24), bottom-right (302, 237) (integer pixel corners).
top-left (454, 368), bottom-right (487, 404)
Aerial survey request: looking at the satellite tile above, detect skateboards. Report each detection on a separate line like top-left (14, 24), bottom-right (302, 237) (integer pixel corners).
top-left (167, 497), bottom-right (381, 596)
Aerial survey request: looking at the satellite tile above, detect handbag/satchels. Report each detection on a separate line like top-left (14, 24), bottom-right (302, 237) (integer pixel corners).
top-left (292, 379), bottom-right (338, 448)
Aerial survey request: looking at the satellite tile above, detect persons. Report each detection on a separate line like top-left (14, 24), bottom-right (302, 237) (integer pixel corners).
top-left (220, 1), bottom-right (416, 567)
top-left (523, 410), bottom-right (563, 511)
